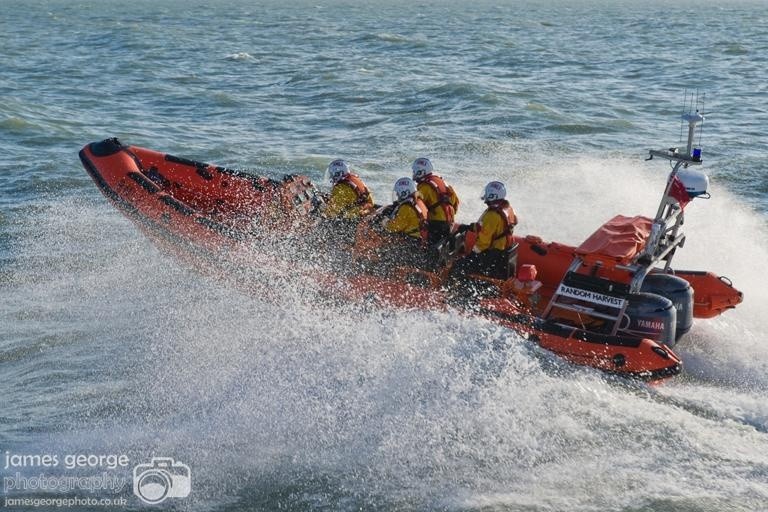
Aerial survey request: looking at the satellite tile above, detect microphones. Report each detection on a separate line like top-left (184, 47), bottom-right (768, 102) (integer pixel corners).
top-left (397, 191), bottom-right (406, 201)
top-left (481, 194), bottom-right (494, 200)
top-left (413, 170), bottom-right (421, 180)
top-left (329, 172), bottom-right (340, 183)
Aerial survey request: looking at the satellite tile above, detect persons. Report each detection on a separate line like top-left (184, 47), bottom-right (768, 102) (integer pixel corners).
top-left (410, 157), bottom-right (460, 245)
top-left (257, 173), bottom-right (328, 250)
top-left (360, 176), bottom-right (430, 280)
top-left (447, 179), bottom-right (519, 303)
top-left (319, 159), bottom-right (374, 247)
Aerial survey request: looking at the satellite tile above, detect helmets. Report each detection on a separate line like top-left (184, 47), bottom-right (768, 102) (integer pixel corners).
top-left (411, 158), bottom-right (433, 181)
top-left (481, 182), bottom-right (506, 203)
top-left (393, 178), bottom-right (417, 200)
top-left (328, 161), bottom-right (350, 186)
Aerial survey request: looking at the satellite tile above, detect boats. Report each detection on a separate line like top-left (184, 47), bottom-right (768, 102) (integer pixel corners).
top-left (74, 82), bottom-right (743, 390)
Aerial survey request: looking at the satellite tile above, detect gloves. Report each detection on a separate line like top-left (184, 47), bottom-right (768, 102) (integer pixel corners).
top-left (464, 250), bottom-right (478, 263)
top-left (457, 224), bottom-right (471, 234)
top-left (382, 201), bottom-right (400, 215)
